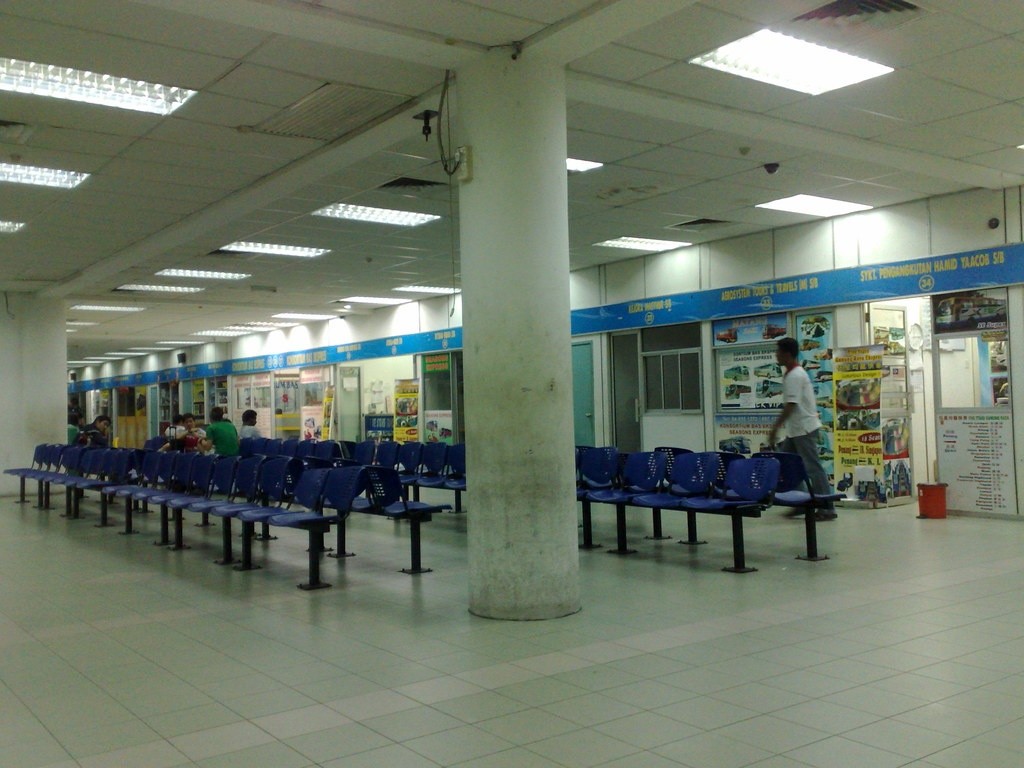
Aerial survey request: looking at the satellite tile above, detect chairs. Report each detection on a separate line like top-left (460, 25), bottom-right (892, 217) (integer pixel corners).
top-left (3, 437), bottom-right (465, 594)
top-left (576, 444), bottom-right (848, 573)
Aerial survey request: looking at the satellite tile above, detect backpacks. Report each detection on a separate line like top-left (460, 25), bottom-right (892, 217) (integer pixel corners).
top-left (75, 430), bottom-right (100, 447)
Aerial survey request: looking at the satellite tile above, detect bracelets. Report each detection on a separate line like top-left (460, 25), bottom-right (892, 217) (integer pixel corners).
top-left (188, 428), bottom-right (192, 433)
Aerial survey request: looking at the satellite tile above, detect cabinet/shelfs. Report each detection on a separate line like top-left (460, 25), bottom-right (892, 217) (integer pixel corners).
top-left (214, 377), bottom-right (229, 419)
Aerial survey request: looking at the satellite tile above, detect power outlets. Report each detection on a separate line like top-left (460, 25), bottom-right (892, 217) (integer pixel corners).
top-left (454, 144), bottom-right (472, 182)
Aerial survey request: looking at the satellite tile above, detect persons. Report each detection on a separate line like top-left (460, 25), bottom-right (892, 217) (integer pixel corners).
top-left (68, 413), bottom-right (111, 447)
top-left (769, 338), bottom-right (838, 521)
top-left (240, 410), bottom-right (262, 440)
top-left (161, 406), bottom-right (240, 457)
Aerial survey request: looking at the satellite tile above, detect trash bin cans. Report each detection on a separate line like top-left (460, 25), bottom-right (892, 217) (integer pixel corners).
top-left (917, 482), bottom-right (949, 519)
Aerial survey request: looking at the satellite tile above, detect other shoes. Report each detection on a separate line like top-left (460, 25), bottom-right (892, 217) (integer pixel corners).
top-left (814, 512), bottom-right (838, 520)
top-left (786, 506), bottom-right (805, 519)
